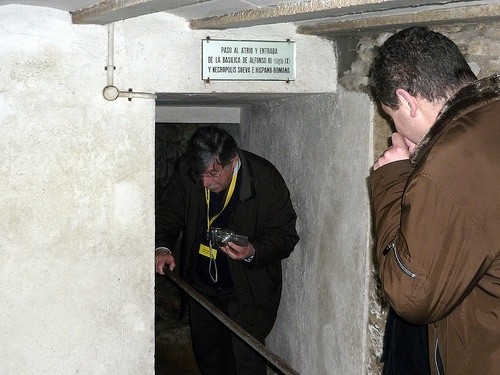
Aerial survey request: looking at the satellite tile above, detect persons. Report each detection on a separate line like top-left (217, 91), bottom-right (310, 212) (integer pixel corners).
top-left (368, 26), bottom-right (500, 375)
top-left (155, 124), bottom-right (301, 375)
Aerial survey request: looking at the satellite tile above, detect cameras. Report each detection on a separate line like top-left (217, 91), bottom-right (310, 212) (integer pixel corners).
top-left (209, 227), bottom-right (248, 250)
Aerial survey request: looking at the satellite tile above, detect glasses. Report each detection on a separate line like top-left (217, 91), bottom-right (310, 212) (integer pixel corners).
top-left (192, 164), bottom-right (226, 182)
